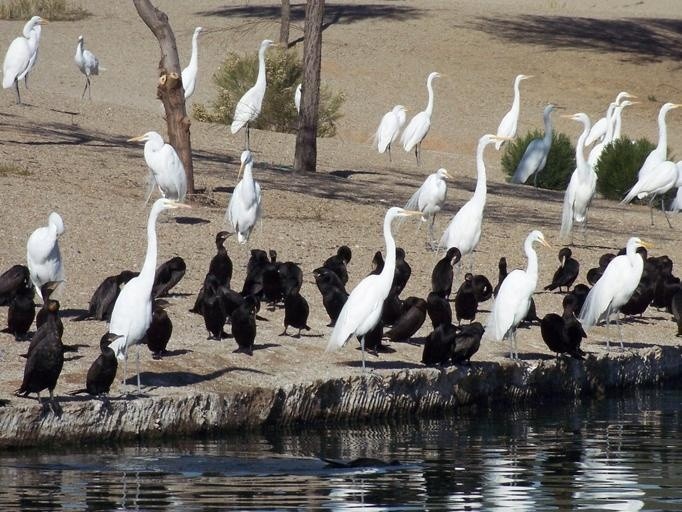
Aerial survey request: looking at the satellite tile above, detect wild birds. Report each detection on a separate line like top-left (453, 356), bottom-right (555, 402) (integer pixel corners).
top-left (435, 133), bottom-right (506, 278)
top-left (369, 101), bottom-right (408, 165)
top-left (556, 112), bottom-right (599, 248)
top-left (75, 34), bottom-right (98, 101)
top-left (0, 197), bottom-right (682, 404)
top-left (394, 166), bottom-right (455, 253)
top-left (635, 100), bottom-right (682, 207)
top-left (125, 130), bottom-right (187, 205)
top-left (624, 163), bottom-right (682, 230)
top-left (398, 71), bottom-right (445, 167)
top-left (583, 85), bottom-right (640, 170)
top-left (181, 26), bottom-right (209, 100)
top-left (493, 75), bottom-right (535, 151)
top-left (231, 38), bottom-right (278, 151)
top-left (509, 103), bottom-right (569, 195)
top-left (2, 15), bottom-right (49, 105)
top-left (227, 149), bottom-right (262, 244)
top-left (295, 83), bottom-right (305, 113)
